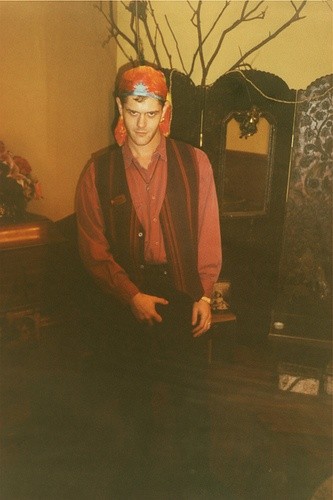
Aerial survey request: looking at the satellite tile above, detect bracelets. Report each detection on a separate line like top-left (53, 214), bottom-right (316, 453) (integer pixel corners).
top-left (201, 296), bottom-right (213, 305)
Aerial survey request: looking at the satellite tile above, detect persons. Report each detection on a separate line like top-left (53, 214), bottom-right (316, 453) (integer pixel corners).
top-left (73, 66), bottom-right (224, 500)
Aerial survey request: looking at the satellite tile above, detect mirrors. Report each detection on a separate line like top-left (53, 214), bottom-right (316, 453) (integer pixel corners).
top-left (216, 111), bottom-right (277, 214)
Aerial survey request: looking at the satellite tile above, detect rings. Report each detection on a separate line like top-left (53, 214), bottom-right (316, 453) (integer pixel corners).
top-left (208, 322), bottom-right (212, 325)
